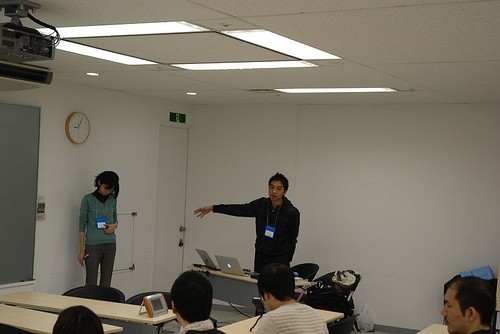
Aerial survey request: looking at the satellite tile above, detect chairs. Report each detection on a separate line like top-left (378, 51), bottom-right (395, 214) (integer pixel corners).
top-left (315, 270), bottom-right (360, 334)
top-left (126, 291), bottom-right (170, 306)
top-left (295, 263), bottom-right (319, 303)
top-left (61, 285), bottom-right (125, 303)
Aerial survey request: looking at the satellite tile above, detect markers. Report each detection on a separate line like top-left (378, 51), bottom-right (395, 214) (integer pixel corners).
top-left (83, 253), bottom-right (89, 259)
top-left (21, 279), bottom-right (36, 282)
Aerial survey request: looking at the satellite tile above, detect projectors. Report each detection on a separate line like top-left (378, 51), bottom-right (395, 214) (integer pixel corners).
top-left (0, 22), bottom-right (55, 63)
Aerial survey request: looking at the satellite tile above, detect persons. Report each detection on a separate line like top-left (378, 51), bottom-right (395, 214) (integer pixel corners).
top-left (253, 262), bottom-right (329, 334)
top-left (440, 276), bottom-right (496, 334)
top-left (170, 270), bottom-right (226, 334)
top-left (77, 170), bottom-right (120, 287)
top-left (194, 172), bottom-right (301, 272)
top-left (53, 305), bottom-right (105, 334)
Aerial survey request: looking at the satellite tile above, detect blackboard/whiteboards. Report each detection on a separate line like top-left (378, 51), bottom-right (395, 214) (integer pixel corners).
top-left (96, 212), bottom-right (137, 275)
top-left (0, 103), bottom-right (42, 289)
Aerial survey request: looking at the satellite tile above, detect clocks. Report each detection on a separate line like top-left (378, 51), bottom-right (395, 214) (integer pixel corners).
top-left (65, 112), bottom-right (89, 145)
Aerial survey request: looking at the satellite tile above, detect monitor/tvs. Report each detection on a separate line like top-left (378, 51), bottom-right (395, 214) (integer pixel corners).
top-left (143, 293), bottom-right (168, 318)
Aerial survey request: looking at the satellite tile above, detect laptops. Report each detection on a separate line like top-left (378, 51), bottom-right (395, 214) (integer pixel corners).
top-left (214, 255), bottom-right (248, 276)
top-left (196, 249), bottom-right (221, 270)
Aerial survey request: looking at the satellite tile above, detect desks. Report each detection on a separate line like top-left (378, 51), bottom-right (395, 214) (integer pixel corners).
top-left (1, 290), bottom-right (176, 326)
top-left (191, 267), bottom-right (310, 308)
top-left (217, 308), bottom-right (345, 334)
top-left (417, 323), bottom-right (450, 334)
top-left (0, 304), bottom-right (123, 334)
